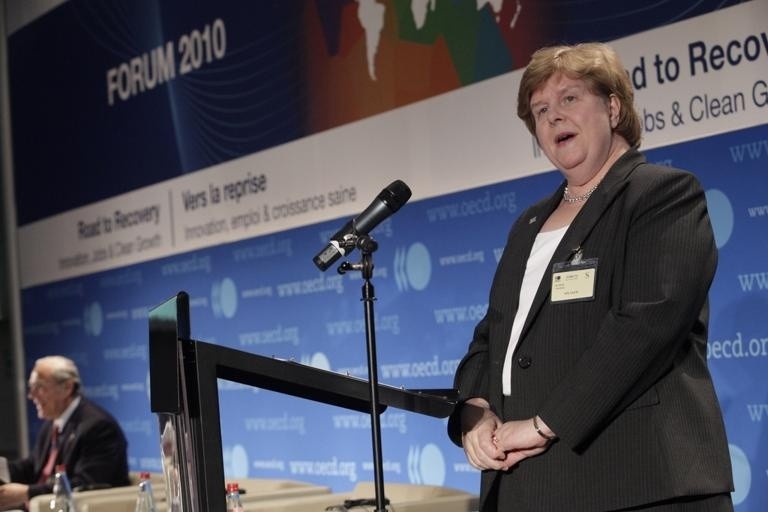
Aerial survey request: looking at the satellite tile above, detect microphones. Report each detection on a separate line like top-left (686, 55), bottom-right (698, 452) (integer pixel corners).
top-left (312, 179), bottom-right (412, 271)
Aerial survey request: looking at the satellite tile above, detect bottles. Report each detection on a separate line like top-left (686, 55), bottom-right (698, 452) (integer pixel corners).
top-left (226, 482), bottom-right (242, 512)
top-left (134, 472), bottom-right (156, 512)
top-left (49, 464), bottom-right (75, 512)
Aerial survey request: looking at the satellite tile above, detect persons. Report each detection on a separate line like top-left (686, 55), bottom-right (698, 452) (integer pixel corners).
top-left (1, 354), bottom-right (129, 511)
top-left (445, 36), bottom-right (740, 511)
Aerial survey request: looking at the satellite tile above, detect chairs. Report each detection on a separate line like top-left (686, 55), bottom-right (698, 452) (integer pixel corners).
top-left (27, 470), bottom-right (480, 512)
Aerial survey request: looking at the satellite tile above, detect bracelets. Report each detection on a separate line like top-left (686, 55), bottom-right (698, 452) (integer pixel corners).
top-left (532, 414), bottom-right (553, 441)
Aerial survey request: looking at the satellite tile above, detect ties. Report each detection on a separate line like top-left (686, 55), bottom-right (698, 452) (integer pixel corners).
top-left (36, 425), bottom-right (60, 485)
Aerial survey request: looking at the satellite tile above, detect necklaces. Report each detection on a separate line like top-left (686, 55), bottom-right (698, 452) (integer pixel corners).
top-left (562, 182), bottom-right (601, 203)
